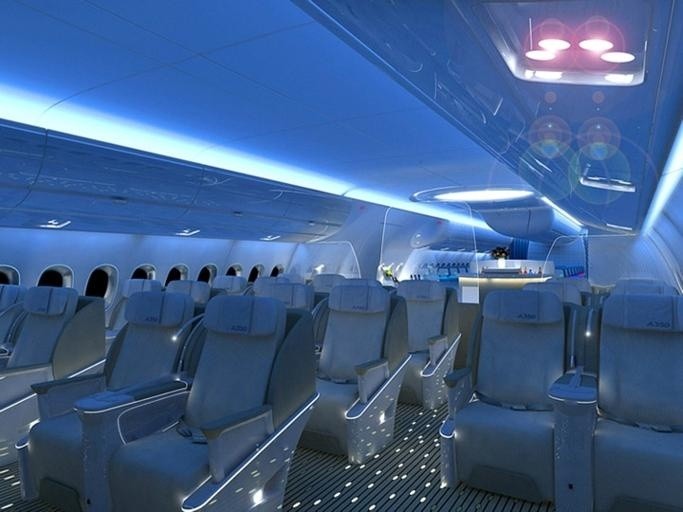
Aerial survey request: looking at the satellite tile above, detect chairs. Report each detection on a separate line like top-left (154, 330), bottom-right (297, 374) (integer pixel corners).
top-left (611, 277), bottom-right (668, 291)
top-left (395, 280), bottom-right (463, 405)
top-left (312, 274), bottom-right (344, 292)
top-left (342, 279), bottom-right (381, 287)
top-left (517, 282), bottom-right (582, 371)
top-left (254, 281), bottom-right (308, 312)
top-left (82, 296), bottom-right (319, 512)
top-left (250, 276), bottom-right (291, 294)
top-left (0, 283), bottom-right (28, 364)
top-left (612, 285), bottom-right (678, 295)
top-left (0, 286), bottom-right (104, 443)
top-left (441, 289), bottom-right (577, 512)
top-left (104, 280), bottom-right (160, 357)
top-left (576, 290), bottom-right (682, 511)
top-left (213, 275), bottom-right (248, 297)
top-left (308, 279), bottom-right (412, 462)
top-left (163, 280), bottom-right (211, 303)
top-left (14, 290), bottom-right (196, 512)
top-left (547, 274), bottom-right (592, 290)
